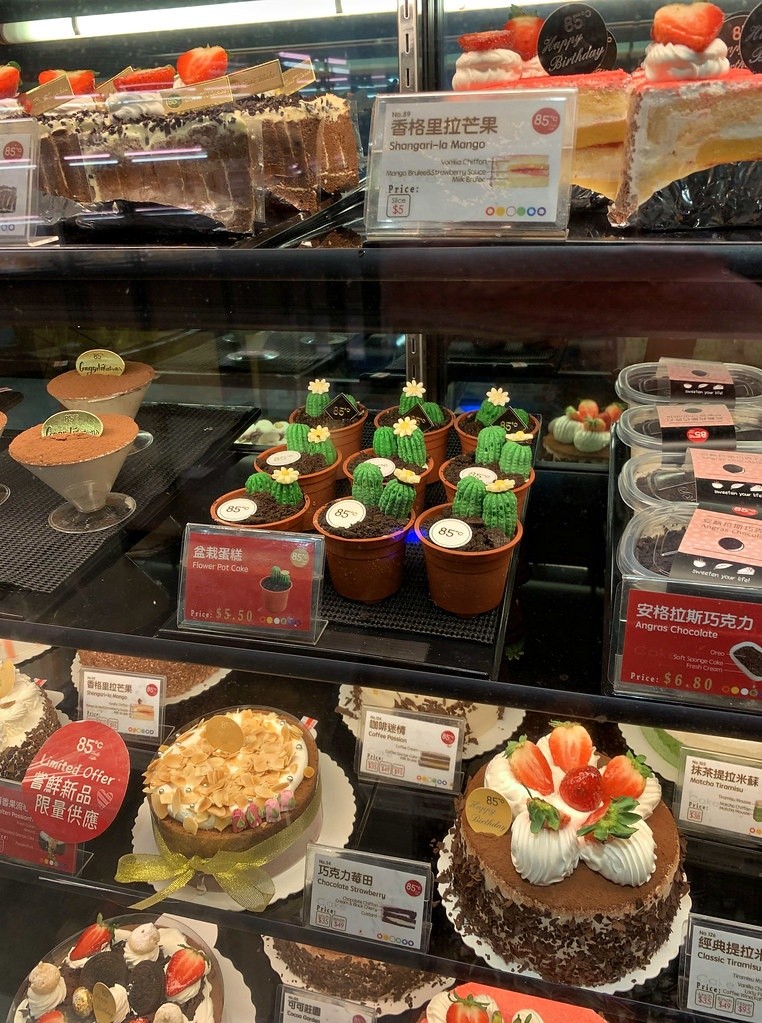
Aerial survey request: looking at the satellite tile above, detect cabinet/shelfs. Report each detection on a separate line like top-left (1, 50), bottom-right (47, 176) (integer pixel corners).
top-left (0, 0), bottom-right (762, 1023)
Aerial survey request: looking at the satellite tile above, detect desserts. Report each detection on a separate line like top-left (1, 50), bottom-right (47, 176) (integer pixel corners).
top-left (1, 1), bottom-right (762, 618)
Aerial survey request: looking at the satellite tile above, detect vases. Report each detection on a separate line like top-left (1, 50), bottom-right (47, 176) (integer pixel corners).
top-left (342, 448), bottom-right (434, 525)
top-left (289, 404), bottom-right (368, 479)
top-left (254, 444), bottom-right (342, 530)
top-left (454, 410), bottom-right (540, 455)
top-left (210, 488), bottom-right (311, 532)
top-left (313, 496), bottom-right (417, 602)
top-left (439, 450), bottom-right (535, 521)
top-left (374, 405), bottom-right (456, 484)
top-left (414, 503), bottom-right (523, 615)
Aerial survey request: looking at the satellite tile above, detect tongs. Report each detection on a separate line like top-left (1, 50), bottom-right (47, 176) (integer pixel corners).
top-left (250, 177), bottom-right (364, 249)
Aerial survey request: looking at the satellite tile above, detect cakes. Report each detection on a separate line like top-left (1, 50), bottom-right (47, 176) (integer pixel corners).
top-left (1, 723), bottom-right (762, 1023)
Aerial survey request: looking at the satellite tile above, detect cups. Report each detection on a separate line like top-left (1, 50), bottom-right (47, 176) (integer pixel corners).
top-left (47, 360), bottom-right (155, 457)
top-left (8, 413), bottom-right (139, 534)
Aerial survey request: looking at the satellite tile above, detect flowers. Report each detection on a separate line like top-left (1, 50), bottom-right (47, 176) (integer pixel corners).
top-left (243, 377), bottom-right (535, 538)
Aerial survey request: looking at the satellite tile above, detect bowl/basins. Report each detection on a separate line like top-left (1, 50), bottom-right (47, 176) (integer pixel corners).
top-left (617, 364), bottom-right (761, 598)
